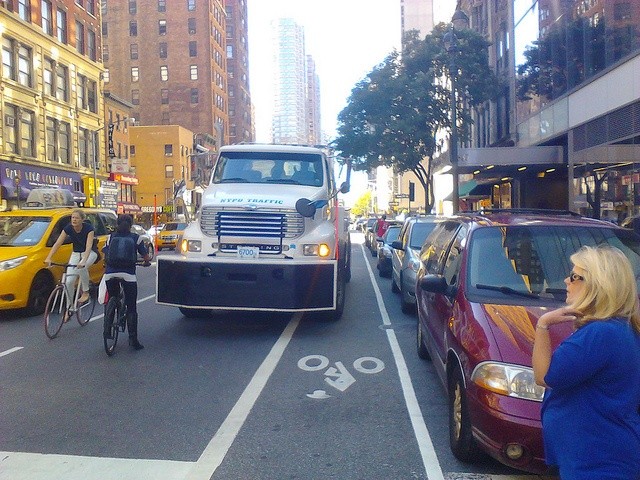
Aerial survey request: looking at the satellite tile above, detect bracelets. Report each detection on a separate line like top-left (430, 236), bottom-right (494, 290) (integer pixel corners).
top-left (536, 324), bottom-right (548, 330)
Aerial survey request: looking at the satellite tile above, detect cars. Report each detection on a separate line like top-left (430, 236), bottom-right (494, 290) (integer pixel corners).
top-left (129, 224), bottom-right (155, 266)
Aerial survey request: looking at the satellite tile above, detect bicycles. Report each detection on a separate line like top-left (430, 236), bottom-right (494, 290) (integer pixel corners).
top-left (42, 262), bottom-right (98, 339)
top-left (102, 261), bottom-right (147, 355)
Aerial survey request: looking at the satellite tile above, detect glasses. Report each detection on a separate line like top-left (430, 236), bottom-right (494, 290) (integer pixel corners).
top-left (569, 271), bottom-right (588, 282)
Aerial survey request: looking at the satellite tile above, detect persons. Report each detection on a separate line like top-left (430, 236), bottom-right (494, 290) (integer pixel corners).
top-left (101, 212), bottom-right (151, 350)
top-left (43, 210), bottom-right (101, 323)
top-left (291, 162), bottom-right (320, 184)
top-left (532, 244), bottom-right (639, 480)
top-left (373, 207), bottom-right (390, 239)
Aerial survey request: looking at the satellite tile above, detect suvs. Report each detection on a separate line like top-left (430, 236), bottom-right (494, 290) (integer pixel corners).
top-left (154, 221), bottom-right (189, 252)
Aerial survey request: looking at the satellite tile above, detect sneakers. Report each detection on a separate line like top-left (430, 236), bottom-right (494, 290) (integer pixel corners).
top-left (77, 295), bottom-right (89, 304)
top-left (62, 311), bottom-right (72, 323)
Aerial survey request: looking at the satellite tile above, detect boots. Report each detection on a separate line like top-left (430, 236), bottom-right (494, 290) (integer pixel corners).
top-left (103, 305), bottom-right (114, 339)
top-left (127, 312), bottom-right (144, 349)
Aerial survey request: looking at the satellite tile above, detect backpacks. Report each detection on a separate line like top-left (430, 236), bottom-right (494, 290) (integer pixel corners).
top-left (108, 231), bottom-right (137, 267)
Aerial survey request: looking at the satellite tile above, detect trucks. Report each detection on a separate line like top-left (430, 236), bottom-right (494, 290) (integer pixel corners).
top-left (154, 141), bottom-right (353, 323)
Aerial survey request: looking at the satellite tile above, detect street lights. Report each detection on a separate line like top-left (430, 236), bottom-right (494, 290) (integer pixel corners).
top-left (444, 1), bottom-right (472, 215)
top-left (13, 176), bottom-right (22, 209)
top-left (91, 117), bottom-right (136, 208)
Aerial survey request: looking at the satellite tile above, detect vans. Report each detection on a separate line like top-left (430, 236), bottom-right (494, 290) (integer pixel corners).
top-left (0, 205), bottom-right (122, 318)
top-left (366, 216), bottom-right (446, 314)
top-left (413, 208), bottom-right (638, 475)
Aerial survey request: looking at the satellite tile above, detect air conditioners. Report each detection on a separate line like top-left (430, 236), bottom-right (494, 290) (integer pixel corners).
top-left (6, 116), bottom-right (15, 127)
